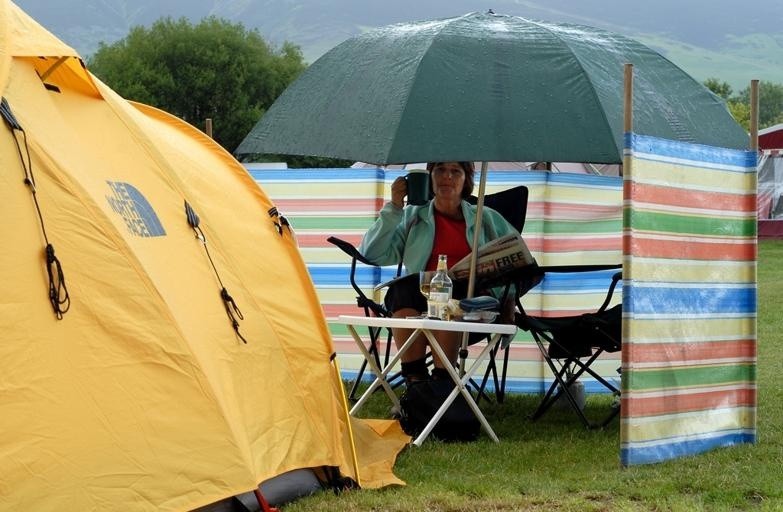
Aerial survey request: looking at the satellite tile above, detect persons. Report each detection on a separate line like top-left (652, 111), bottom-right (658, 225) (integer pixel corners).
top-left (359, 159), bottom-right (522, 443)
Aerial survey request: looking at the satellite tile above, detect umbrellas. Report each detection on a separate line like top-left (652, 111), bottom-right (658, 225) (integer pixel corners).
top-left (230, 4), bottom-right (754, 322)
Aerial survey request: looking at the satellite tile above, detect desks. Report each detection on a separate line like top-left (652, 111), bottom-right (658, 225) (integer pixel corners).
top-left (327, 314), bottom-right (517, 448)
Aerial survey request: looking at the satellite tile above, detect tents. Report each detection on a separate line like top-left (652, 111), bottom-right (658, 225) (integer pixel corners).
top-left (1, 0), bottom-right (414, 511)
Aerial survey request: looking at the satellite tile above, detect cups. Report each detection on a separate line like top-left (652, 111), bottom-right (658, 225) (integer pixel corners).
top-left (418, 270), bottom-right (436, 300)
top-left (402, 169), bottom-right (430, 206)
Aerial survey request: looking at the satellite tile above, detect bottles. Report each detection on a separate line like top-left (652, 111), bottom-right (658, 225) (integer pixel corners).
top-left (429, 254), bottom-right (454, 302)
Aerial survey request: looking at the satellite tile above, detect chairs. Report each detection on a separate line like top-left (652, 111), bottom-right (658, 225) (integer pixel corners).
top-left (513, 271), bottom-right (622, 435)
top-left (326, 185), bottom-right (531, 406)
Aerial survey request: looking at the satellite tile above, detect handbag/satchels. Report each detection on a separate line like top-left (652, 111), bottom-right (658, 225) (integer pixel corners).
top-left (399, 379), bottom-right (481, 442)
